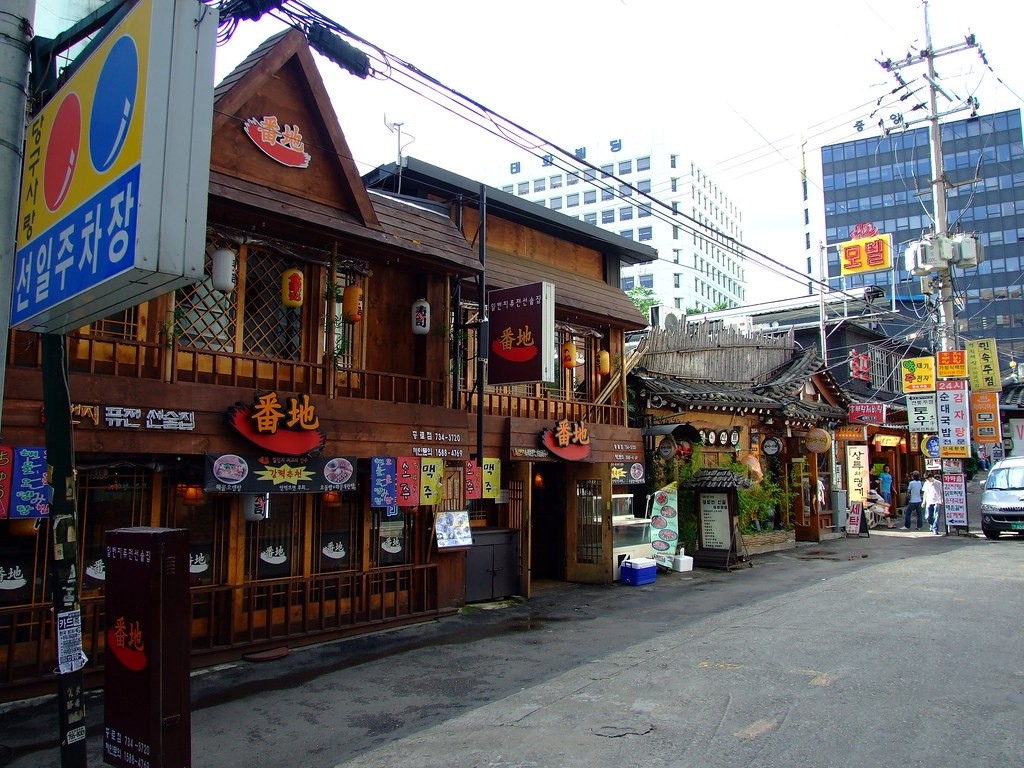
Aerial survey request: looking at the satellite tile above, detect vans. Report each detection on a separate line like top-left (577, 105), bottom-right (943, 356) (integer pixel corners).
top-left (979, 458), bottom-right (1024, 538)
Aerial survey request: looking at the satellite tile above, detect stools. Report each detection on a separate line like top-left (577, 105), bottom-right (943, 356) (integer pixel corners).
top-left (863, 507), bottom-right (884, 527)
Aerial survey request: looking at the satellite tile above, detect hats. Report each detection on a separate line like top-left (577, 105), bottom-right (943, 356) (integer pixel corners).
top-left (910, 470), bottom-right (920, 476)
top-left (924, 472), bottom-right (934, 479)
top-left (979, 446), bottom-right (983, 448)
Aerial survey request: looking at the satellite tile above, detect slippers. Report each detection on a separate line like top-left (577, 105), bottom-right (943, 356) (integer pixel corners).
top-left (885, 514), bottom-right (893, 517)
top-left (888, 524), bottom-right (896, 529)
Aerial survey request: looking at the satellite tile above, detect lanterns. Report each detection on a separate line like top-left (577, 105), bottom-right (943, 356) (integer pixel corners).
top-left (211, 248), bottom-right (236, 294)
top-left (281, 268), bottom-right (304, 310)
top-left (596, 349), bottom-right (609, 375)
top-left (562, 341), bottom-right (576, 371)
top-left (342, 285), bottom-right (364, 325)
top-left (244, 494), bottom-right (264, 522)
top-left (411, 298), bottom-right (431, 334)
top-left (875, 440), bottom-right (882, 453)
top-left (901, 444), bottom-right (906, 454)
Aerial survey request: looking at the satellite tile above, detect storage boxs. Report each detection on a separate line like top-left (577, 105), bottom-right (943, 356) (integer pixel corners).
top-left (620, 557), bottom-right (656, 586)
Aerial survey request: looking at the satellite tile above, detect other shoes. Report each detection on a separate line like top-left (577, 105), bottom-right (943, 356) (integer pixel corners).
top-left (930, 528), bottom-right (938, 535)
top-left (900, 526), bottom-right (910, 530)
top-left (915, 527), bottom-right (921, 531)
top-left (984, 468), bottom-right (991, 470)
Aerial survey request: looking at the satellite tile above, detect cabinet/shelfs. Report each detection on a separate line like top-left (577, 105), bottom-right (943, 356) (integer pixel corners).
top-left (831, 489), bottom-right (848, 534)
top-left (576, 494), bottom-right (652, 581)
top-left (465, 526), bottom-right (520, 602)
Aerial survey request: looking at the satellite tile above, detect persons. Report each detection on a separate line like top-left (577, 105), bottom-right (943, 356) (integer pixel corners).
top-left (921, 472), bottom-right (943, 535)
top-left (899, 471), bottom-right (923, 530)
top-left (867, 482), bottom-right (896, 528)
top-left (869, 463), bottom-right (884, 499)
top-left (976, 445), bottom-right (991, 470)
top-left (876, 465), bottom-right (898, 506)
